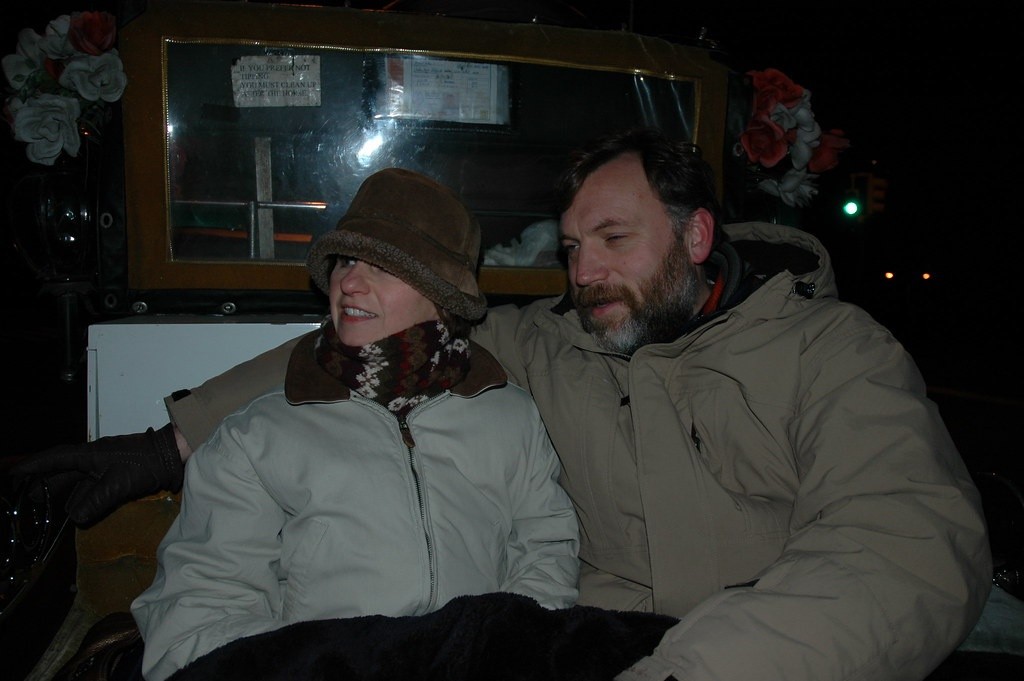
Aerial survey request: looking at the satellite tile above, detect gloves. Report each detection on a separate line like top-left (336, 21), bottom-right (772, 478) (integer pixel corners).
top-left (9, 422), bottom-right (184, 528)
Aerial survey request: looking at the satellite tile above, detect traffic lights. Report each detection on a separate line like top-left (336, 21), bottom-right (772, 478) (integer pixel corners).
top-left (841, 196), bottom-right (864, 224)
top-left (870, 179), bottom-right (888, 214)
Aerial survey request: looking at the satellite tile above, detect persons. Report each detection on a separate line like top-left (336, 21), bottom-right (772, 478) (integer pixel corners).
top-left (129, 168), bottom-right (581, 681)
top-left (0, 130), bottom-right (997, 681)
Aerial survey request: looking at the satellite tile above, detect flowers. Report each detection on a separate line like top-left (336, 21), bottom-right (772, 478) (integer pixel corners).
top-left (0, 10), bottom-right (128, 167)
top-left (736, 66), bottom-right (852, 211)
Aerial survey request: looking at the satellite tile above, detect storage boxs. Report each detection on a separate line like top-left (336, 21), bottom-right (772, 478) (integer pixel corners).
top-left (384, 56), bottom-right (511, 126)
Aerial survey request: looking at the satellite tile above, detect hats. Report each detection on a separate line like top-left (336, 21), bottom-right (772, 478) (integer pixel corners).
top-left (306, 168), bottom-right (487, 323)
